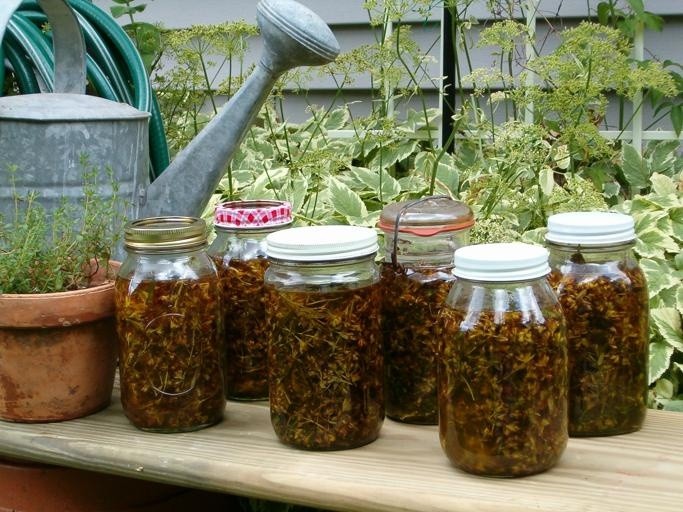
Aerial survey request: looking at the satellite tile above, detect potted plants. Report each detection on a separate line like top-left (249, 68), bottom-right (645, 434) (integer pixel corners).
top-left (0, 148), bottom-right (137, 421)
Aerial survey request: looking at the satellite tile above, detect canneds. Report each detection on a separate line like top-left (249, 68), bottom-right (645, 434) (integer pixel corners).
top-left (205, 200), bottom-right (298, 403)
top-left (262, 224), bottom-right (386, 451)
top-left (113, 216), bottom-right (228, 434)
top-left (543, 211), bottom-right (649, 438)
top-left (377, 202), bottom-right (476, 427)
top-left (437, 243), bottom-right (569, 478)
